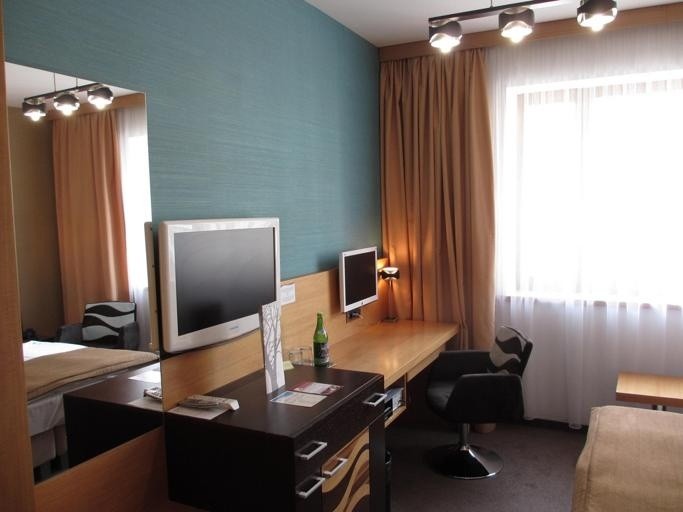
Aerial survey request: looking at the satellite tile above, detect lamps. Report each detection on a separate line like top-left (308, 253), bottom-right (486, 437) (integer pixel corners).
top-left (22, 73), bottom-right (115, 123)
top-left (426, 1), bottom-right (625, 55)
top-left (380, 268), bottom-right (400, 323)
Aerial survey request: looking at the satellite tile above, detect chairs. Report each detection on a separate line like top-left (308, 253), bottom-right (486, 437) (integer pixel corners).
top-left (59, 302), bottom-right (137, 348)
top-left (424, 326), bottom-right (533, 481)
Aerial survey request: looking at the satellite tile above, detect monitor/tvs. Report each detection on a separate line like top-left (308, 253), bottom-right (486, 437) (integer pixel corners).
top-left (159, 217), bottom-right (281, 354)
top-left (144, 222), bottom-right (159, 353)
top-left (339, 246), bottom-right (378, 313)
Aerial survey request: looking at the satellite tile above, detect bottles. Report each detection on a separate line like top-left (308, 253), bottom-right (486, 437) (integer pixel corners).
top-left (314, 312), bottom-right (329, 366)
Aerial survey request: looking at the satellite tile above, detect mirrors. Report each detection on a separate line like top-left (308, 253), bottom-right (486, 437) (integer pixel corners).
top-left (4, 63), bottom-right (163, 484)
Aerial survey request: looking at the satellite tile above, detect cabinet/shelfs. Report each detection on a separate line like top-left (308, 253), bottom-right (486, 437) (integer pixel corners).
top-left (62, 362), bottom-right (164, 465)
top-left (166, 361), bottom-right (388, 510)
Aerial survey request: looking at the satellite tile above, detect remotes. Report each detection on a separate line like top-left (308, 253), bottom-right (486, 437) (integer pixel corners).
top-left (179, 394), bottom-right (240, 411)
top-left (144, 386), bottom-right (162, 399)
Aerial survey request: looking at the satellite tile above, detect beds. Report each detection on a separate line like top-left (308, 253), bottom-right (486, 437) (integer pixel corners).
top-left (570, 403), bottom-right (681, 511)
top-left (19, 339), bottom-right (158, 477)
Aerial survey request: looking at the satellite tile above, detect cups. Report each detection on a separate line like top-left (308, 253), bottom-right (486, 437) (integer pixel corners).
top-left (289, 345), bottom-right (312, 367)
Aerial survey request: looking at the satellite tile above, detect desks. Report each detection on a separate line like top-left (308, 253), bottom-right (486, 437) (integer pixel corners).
top-left (288, 315), bottom-right (459, 423)
top-left (612, 369), bottom-right (682, 411)
